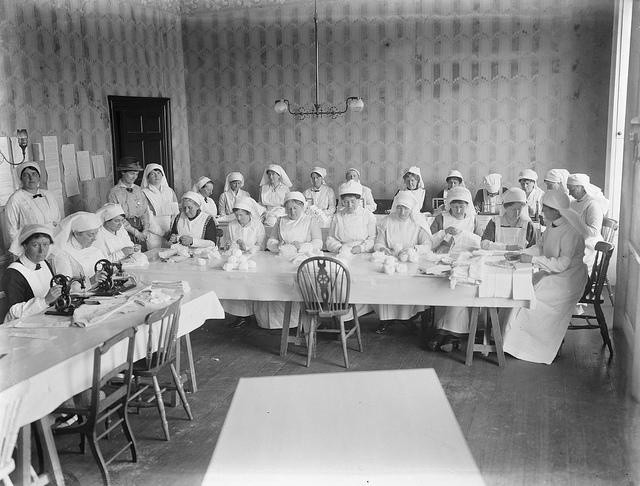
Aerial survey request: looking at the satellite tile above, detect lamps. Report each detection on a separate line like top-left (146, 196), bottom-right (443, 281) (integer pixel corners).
top-left (272, 2), bottom-right (365, 121)
top-left (0, 127), bottom-right (30, 167)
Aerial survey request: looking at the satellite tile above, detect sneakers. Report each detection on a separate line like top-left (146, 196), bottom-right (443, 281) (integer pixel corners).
top-left (376, 322), bottom-right (390, 333)
top-left (428, 336), bottom-right (463, 353)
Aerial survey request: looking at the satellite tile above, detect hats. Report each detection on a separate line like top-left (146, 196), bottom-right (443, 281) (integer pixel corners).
top-left (6, 221), bottom-right (55, 257)
top-left (311, 166), bottom-right (327, 180)
top-left (345, 167), bottom-right (360, 177)
top-left (446, 184), bottom-right (469, 208)
top-left (401, 167), bottom-right (423, 181)
top-left (232, 197), bottom-right (265, 218)
top-left (282, 191), bottom-right (308, 207)
top-left (257, 163), bottom-right (293, 188)
top-left (335, 180), bottom-right (363, 198)
top-left (181, 192), bottom-right (203, 208)
top-left (223, 171), bottom-right (244, 192)
top-left (68, 210), bottom-right (102, 233)
top-left (117, 156), bottom-right (144, 174)
top-left (143, 162), bottom-right (166, 179)
top-left (390, 190), bottom-right (419, 214)
top-left (483, 167), bottom-right (591, 213)
top-left (95, 203), bottom-right (126, 224)
top-left (445, 169), bottom-right (464, 185)
top-left (16, 161), bottom-right (41, 181)
top-left (193, 175), bottom-right (212, 191)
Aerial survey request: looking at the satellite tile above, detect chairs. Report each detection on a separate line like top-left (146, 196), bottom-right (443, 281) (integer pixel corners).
top-left (555, 217), bottom-right (616, 356)
top-left (31, 325), bottom-right (139, 486)
top-left (105, 295), bottom-right (195, 442)
top-left (297, 255), bottom-right (364, 369)
top-left (432, 199), bottom-right (445, 210)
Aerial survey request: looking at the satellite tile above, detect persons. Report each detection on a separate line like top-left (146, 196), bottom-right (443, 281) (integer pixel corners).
top-left (165, 188), bottom-right (218, 250)
top-left (302, 166), bottom-right (337, 217)
top-left (253, 190), bottom-right (323, 333)
top-left (425, 187), bottom-right (485, 355)
top-left (258, 164), bottom-right (296, 208)
top-left (479, 185), bottom-right (537, 250)
top-left (492, 189), bottom-right (595, 366)
top-left (218, 171), bottom-right (251, 218)
top-left (97, 202), bottom-right (147, 270)
top-left (517, 169), bottom-right (547, 219)
top-left (191, 175), bottom-right (218, 222)
top-left (344, 166), bottom-right (378, 212)
top-left (105, 155), bottom-right (153, 252)
top-left (564, 172), bottom-right (611, 288)
top-left (3, 161), bottom-right (61, 258)
top-left (1, 221), bottom-right (83, 429)
top-left (432, 170), bottom-right (466, 207)
top-left (473, 172), bottom-right (513, 215)
top-left (324, 178), bottom-right (375, 321)
top-left (46, 207), bottom-right (112, 293)
top-left (372, 187), bottom-right (433, 337)
top-left (222, 194), bottom-right (268, 328)
top-left (392, 164), bottom-right (429, 214)
top-left (543, 168), bottom-right (573, 203)
top-left (139, 162), bottom-right (181, 248)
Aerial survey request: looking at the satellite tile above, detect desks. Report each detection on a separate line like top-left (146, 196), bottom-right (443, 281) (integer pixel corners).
top-left (0, 281), bottom-right (220, 484)
top-left (215, 214), bottom-right (549, 242)
top-left (113, 243), bottom-right (534, 364)
top-left (202, 366), bottom-right (482, 485)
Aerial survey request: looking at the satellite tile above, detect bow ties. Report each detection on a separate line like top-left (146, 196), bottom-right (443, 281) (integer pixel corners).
top-left (33, 193), bottom-right (42, 199)
